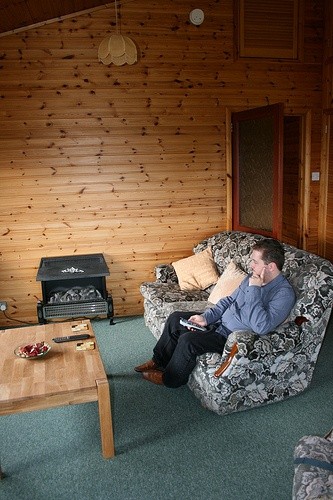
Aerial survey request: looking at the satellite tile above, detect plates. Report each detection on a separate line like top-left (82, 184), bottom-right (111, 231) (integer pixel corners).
top-left (14, 342), bottom-right (52, 359)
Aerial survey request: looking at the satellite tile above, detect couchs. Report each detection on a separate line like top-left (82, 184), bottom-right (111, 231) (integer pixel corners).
top-left (140, 231), bottom-right (333, 416)
top-left (292, 424), bottom-right (333, 500)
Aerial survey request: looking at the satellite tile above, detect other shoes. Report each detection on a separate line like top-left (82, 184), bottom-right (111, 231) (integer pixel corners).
top-left (143, 371), bottom-right (164, 385)
top-left (134, 360), bottom-right (156, 372)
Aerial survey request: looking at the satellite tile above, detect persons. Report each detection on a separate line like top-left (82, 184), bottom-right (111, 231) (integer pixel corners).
top-left (135, 239), bottom-right (295, 388)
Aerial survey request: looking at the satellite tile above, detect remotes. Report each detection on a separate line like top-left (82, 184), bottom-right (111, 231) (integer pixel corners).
top-left (180, 318), bottom-right (207, 332)
top-left (54, 334), bottom-right (90, 343)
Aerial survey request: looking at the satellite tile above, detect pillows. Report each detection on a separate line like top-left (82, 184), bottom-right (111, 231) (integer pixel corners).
top-left (208, 259), bottom-right (248, 305)
top-left (172, 248), bottom-right (220, 290)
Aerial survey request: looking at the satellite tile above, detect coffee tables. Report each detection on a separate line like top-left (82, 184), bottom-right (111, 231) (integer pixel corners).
top-left (0, 319), bottom-right (114, 458)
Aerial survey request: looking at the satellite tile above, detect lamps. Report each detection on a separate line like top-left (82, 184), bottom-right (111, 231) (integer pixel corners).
top-left (98, 0), bottom-right (137, 66)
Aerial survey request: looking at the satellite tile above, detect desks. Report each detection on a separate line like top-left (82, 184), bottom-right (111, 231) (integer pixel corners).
top-left (36, 253), bottom-right (116, 325)
